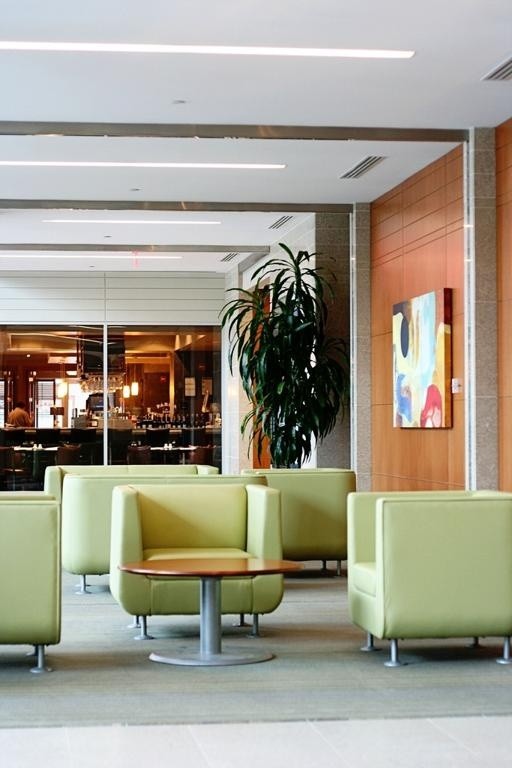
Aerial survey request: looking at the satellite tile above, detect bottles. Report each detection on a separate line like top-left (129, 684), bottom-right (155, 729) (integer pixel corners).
top-left (117, 411), bottom-right (215, 430)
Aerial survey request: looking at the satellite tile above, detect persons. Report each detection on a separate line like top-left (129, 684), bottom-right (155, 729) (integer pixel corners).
top-left (8, 401), bottom-right (37, 426)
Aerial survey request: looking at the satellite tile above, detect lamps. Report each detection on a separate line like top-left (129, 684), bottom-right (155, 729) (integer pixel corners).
top-left (57, 362), bottom-right (139, 399)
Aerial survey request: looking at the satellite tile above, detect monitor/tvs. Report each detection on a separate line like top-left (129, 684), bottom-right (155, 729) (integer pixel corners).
top-left (83, 338), bottom-right (124, 372)
top-left (87, 393), bottom-right (109, 410)
